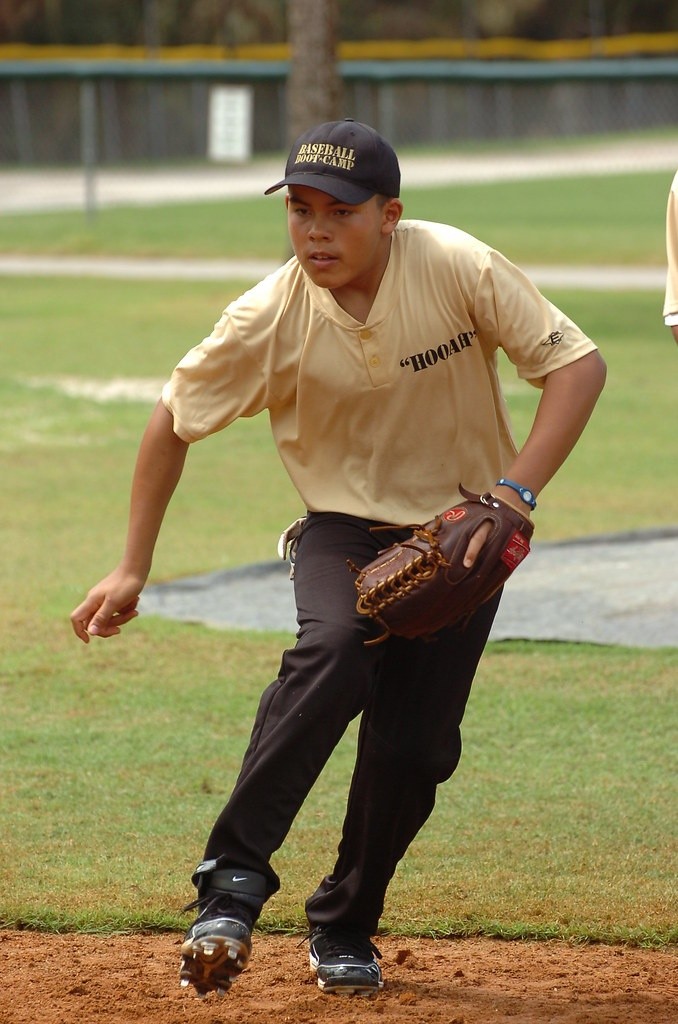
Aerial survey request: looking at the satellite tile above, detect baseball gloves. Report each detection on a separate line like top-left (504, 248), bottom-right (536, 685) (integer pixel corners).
top-left (346, 482), bottom-right (536, 649)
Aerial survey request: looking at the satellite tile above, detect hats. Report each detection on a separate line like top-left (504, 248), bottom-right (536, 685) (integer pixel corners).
top-left (263, 117), bottom-right (402, 209)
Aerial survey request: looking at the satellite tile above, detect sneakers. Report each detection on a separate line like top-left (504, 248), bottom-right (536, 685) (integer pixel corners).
top-left (175, 894), bottom-right (253, 1000)
top-left (298, 922), bottom-right (384, 998)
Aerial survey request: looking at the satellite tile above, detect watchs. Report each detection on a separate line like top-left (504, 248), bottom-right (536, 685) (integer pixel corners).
top-left (496, 478), bottom-right (536, 511)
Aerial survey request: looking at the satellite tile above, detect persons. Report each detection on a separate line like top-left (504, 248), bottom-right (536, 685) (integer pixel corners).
top-left (69, 118), bottom-right (607, 995)
